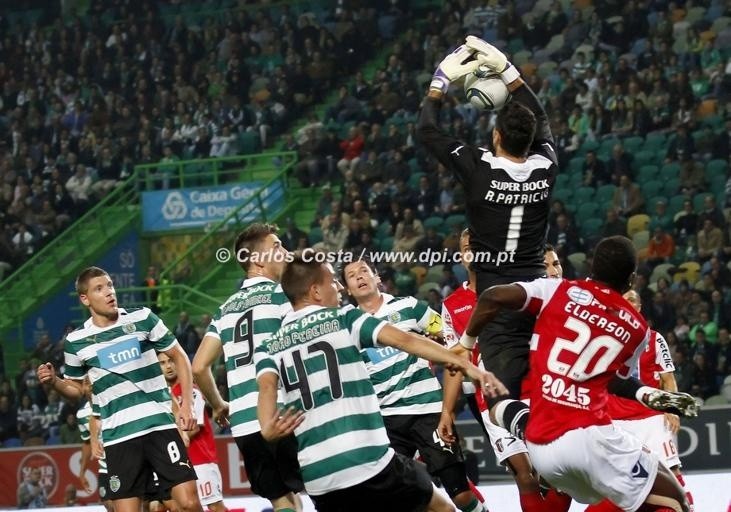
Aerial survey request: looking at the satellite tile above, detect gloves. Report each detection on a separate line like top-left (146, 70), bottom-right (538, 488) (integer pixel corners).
top-left (466, 35), bottom-right (508, 74)
top-left (430, 43), bottom-right (487, 94)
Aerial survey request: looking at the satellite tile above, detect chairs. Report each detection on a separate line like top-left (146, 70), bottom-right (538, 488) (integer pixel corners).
top-left (312, 118), bottom-right (467, 298)
top-left (549, 127), bottom-right (729, 234)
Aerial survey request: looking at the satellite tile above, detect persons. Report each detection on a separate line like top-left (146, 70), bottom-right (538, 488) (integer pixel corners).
top-left (75, 349), bottom-right (232, 510)
top-left (422, 35), bottom-right (704, 492)
top-left (34, 266), bottom-right (204, 511)
top-left (0, 0), bottom-right (731, 451)
top-left (189, 220), bottom-right (375, 512)
top-left (15, 466), bottom-right (49, 507)
top-left (431, 226), bottom-right (695, 512)
top-left (252, 248), bottom-right (511, 511)
top-left (412, 426), bottom-right (491, 512)
top-left (336, 248), bottom-right (492, 512)
top-left (62, 484), bottom-right (89, 506)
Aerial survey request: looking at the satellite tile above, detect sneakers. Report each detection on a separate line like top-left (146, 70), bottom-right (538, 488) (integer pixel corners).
top-left (642, 389), bottom-right (701, 419)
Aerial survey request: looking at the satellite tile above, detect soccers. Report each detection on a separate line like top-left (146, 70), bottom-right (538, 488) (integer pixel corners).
top-left (463, 64), bottom-right (513, 111)
